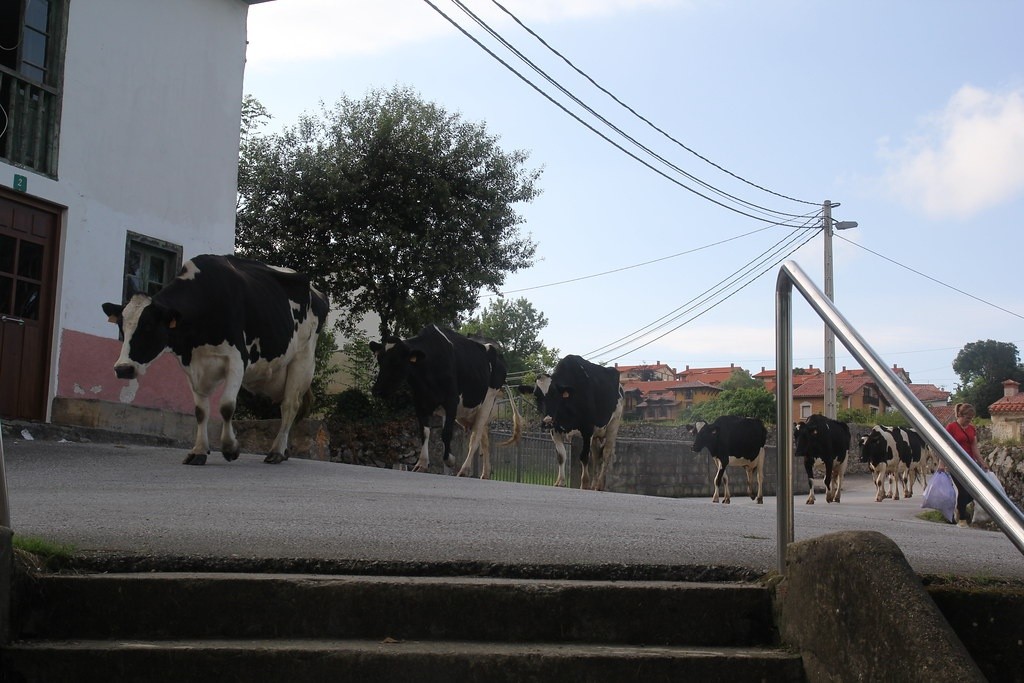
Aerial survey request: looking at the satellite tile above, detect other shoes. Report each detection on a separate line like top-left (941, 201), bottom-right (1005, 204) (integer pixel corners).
top-left (956, 523), bottom-right (968, 528)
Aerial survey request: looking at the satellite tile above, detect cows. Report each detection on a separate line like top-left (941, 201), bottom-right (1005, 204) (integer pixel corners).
top-left (685, 415), bottom-right (768, 505)
top-left (532, 354), bottom-right (627, 491)
top-left (368, 324), bottom-right (522, 480)
top-left (858, 427), bottom-right (934, 502)
top-left (793, 413), bottom-right (850, 505)
top-left (102, 253), bottom-right (329, 466)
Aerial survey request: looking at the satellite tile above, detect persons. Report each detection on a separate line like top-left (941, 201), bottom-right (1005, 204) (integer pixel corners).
top-left (937, 401), bottom-right (989, 528)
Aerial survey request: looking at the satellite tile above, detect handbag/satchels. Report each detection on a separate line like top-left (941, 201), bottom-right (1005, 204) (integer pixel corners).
top-left (972, 470), bottom-right (1007, 523)
top-left (920, 469), bottom-right (956, 522)
